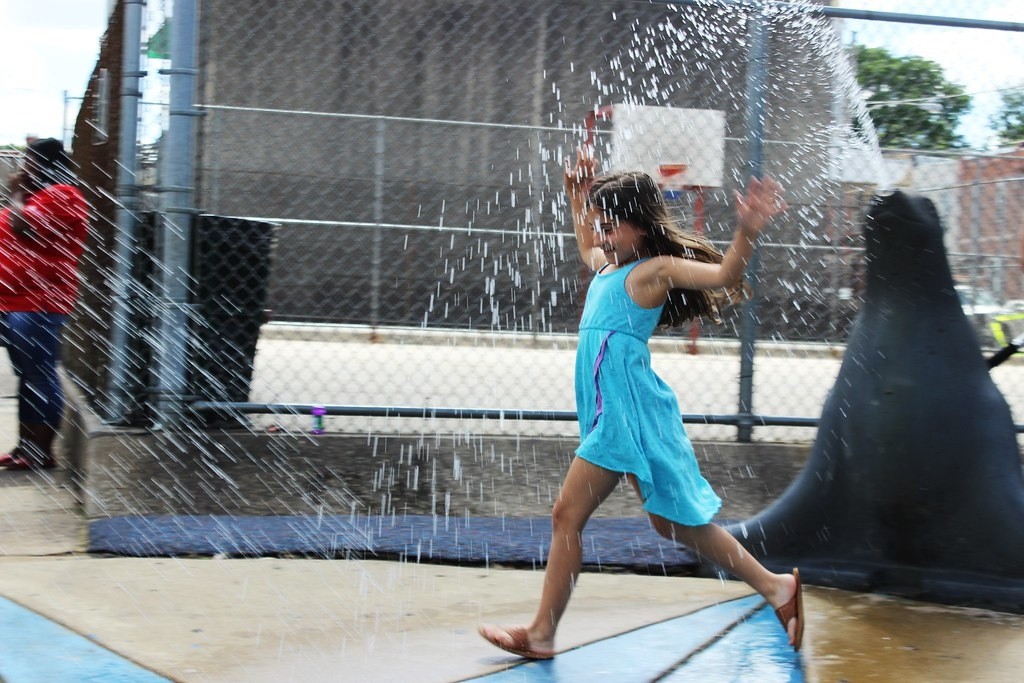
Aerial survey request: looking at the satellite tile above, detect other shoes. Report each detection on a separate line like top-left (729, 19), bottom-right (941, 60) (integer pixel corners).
top-left (0, 447), bottom-right (56, 469)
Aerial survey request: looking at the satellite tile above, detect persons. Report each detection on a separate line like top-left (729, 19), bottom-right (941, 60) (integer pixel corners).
top-left (481, 140), bottom-right (807, 661)
top-left (0, 140), bottom-right (89, 474)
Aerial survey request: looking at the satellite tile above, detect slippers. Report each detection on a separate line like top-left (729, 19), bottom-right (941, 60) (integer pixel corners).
top-left (776, 567), bottom-right (804, 654)
top-left (478, 625), bottom-right (554, 660)
